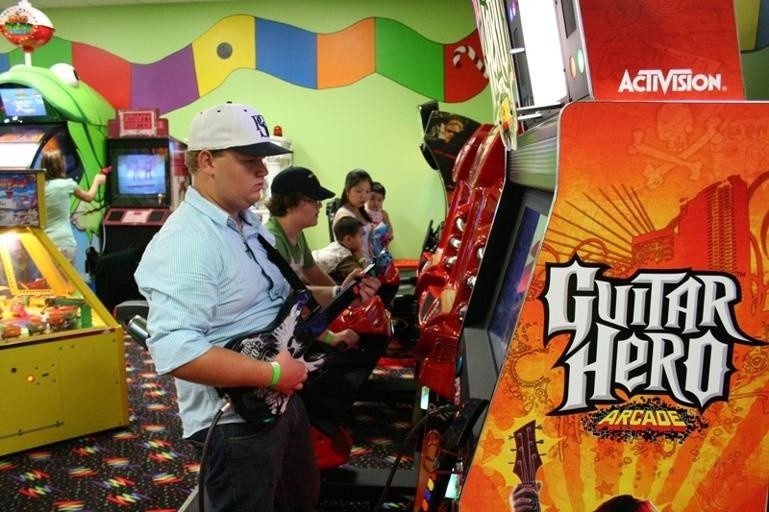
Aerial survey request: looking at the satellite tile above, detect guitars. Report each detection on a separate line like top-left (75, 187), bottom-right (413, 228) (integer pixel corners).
top-left (215, 247), bottom-right (392, 426)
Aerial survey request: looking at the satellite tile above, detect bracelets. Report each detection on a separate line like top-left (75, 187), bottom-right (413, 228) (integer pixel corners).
top-left (323, 329), bottom-right (335, 344)
top-left (269, 360), bottom-right (282, 390)
top-left (332, 284), bottom-right (340, 301)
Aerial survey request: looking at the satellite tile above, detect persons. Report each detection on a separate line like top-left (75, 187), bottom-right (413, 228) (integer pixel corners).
top-left (366, 181), bottom-right (394, 249)
top-left (311, 216), bottom-right (366, 277)
top-left (263, 165), bottom-right (389, 436)
top-left (133, 101), bottom-right (382, 511)
top-left (330, 168), bottom-right (399, 307)
top-left (37, 150), bottom-right (107, 266)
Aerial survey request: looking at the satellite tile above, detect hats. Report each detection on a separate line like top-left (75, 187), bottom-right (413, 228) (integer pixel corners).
top-left (186, 100), bottom-right (293, 156)
top-left (271, 166), bottom-right (335, 200)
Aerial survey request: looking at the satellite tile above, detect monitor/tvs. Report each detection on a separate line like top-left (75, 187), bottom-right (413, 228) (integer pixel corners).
top-left (108, 146), bottom-right (168, 207)
top-left (481, 191), bottom-right (552, 377)
